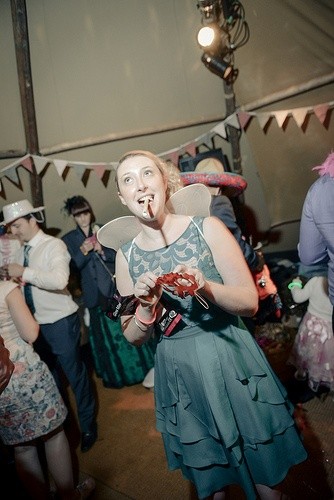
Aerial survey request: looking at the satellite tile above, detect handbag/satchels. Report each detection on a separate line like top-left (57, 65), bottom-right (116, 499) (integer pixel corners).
top-left (109, 275), bottom-right (116, 298)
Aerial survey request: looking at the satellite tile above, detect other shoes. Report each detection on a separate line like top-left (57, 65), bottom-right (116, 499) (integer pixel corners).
top-left (76, 478), bottom-right (96, 500)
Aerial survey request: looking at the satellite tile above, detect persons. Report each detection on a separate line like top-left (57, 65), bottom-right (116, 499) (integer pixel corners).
top-left (289, 145), bottom-right (334, 396)
top-left (188, 156), bottom-right (284, 353)
top-left (114, 150), bottom-right (311, 499)
top-left (52, 195), bottom-right (157, 389)
top-left (0, 274), bottom-right (96, 500)
top-left (3, 198), bottom-right (97, 451)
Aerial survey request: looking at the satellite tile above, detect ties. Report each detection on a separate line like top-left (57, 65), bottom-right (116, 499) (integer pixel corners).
top-left (23, 244), bottom-right (35, 316)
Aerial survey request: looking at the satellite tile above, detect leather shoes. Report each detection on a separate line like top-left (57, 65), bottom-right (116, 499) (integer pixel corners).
top-left (80, 421), bottom-right (97, 452)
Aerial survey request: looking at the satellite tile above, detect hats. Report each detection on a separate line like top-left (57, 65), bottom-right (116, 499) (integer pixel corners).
top-left (0, 200), bottom-right (46, 226)
top-left (179, 158), bottom-right (247, 197)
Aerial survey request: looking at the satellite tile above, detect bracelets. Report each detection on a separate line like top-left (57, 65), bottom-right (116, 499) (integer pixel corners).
top-left (135, 304), bottom-right (157, 325)
top-left (289, 282), bottom-right (301, 289)
top-left (134, 315), bottom-right (152, 333)
